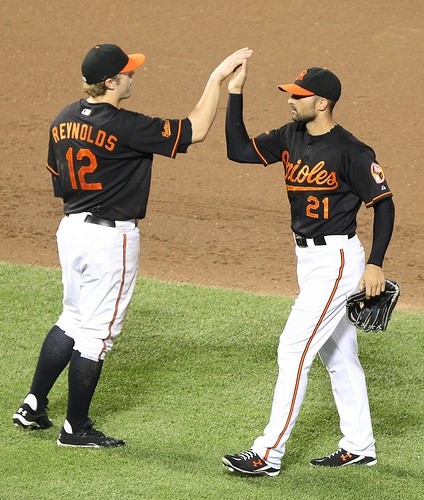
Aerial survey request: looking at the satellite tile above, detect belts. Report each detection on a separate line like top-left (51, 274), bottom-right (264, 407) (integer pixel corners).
top-left (295, 232), bottom-right (356, 247)
top-left (84, 216), bottom-right (138, 229)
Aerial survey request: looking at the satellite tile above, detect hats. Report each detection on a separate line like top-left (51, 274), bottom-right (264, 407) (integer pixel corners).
top-left (278, 66), bottom-right (341, 101)
top-left (82, 44), bottom-right (145, 85)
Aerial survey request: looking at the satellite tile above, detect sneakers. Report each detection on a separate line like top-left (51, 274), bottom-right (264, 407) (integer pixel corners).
top-left (57, 420), bottom-right (126, 447)
top-left (309, 448), bottom-right (377, 467)
top-left (222, 449), bottom-right (280, 476)
top-left (13, 393), bottom-right (52, 429)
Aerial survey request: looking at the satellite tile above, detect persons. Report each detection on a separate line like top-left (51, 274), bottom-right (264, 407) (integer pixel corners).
top-left (11, 43), bottom-right (253, 448)
top-left (222, 59), bottom-right (396, 476)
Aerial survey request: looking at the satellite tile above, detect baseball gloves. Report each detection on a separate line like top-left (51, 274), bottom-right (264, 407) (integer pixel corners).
top-left (345, 279), bottom-right (401, 334)
